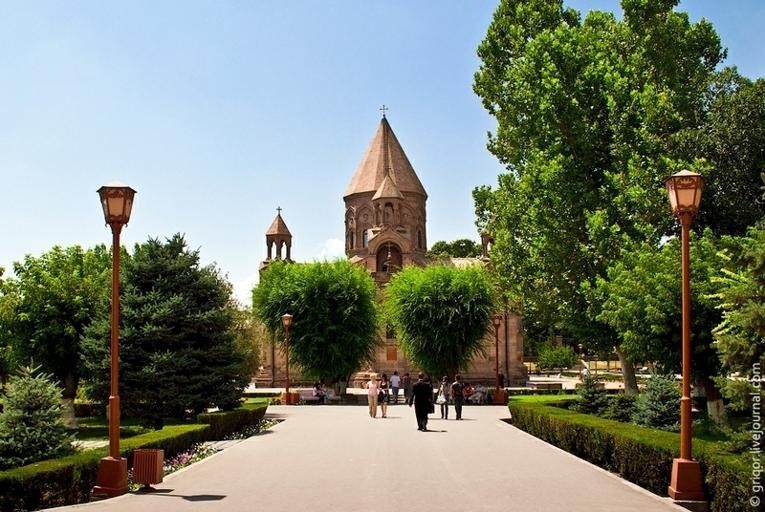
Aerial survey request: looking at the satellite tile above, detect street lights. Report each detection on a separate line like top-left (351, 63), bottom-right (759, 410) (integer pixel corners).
top-left (491, 312), bottom-right (508, 406)
top-left (577, 344), bottom-right (583, 380)
top-left (280, 312), bottom-right (293, 404)
top-left (656, 168), bottom-right (710, 512)
top-left (87, 186), bottom-right (137, 499)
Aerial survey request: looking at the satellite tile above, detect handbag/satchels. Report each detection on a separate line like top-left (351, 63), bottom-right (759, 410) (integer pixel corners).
top-left (436, 394), bottom-right (446, 404)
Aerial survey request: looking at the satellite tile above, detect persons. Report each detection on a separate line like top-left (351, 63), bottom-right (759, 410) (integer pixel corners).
top-left (363, 373), bottom-right (382, 419)
top-left (462, 383), bottom-right (473, 402)
top-left (449, 373), bottom-right (466, 420)
top-left (582, 367), bottom-right (588, 382)
top-left (498, 369), bottom-right (505, 389)
top-left (408, 372), bottom-right (432, 432)
top-left (378, 373), bottom-right (390, 419)
top-left (312, 383), bottom-right (327, 405)
top-left (424, 377), bottom-right (434, 421)
top-left (402, 373), bottom-right (413, 405)
top-left (437, 376), bottom-right (451, 419)
top-left (319, 383), bottom-right (341, 401)
top-left (468, 383), bottom-right (486, 405)
top-left (390, 371), bottom-right (401, 405)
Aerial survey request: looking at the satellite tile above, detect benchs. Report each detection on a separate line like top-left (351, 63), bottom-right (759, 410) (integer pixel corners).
top-left (296, 390), bottom-right (342, 404)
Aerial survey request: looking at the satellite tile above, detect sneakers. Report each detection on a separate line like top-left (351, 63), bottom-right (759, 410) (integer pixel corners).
top-left (369, 412), bottom-right (387, 418)
top-left (394, 398), bottom-right (408, 404)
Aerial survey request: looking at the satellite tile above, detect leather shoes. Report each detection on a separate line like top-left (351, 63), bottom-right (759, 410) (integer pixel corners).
top-left (418, 422), bottom-right (426, 430)
top-left (442, 416), bottom-right (461, 419)
